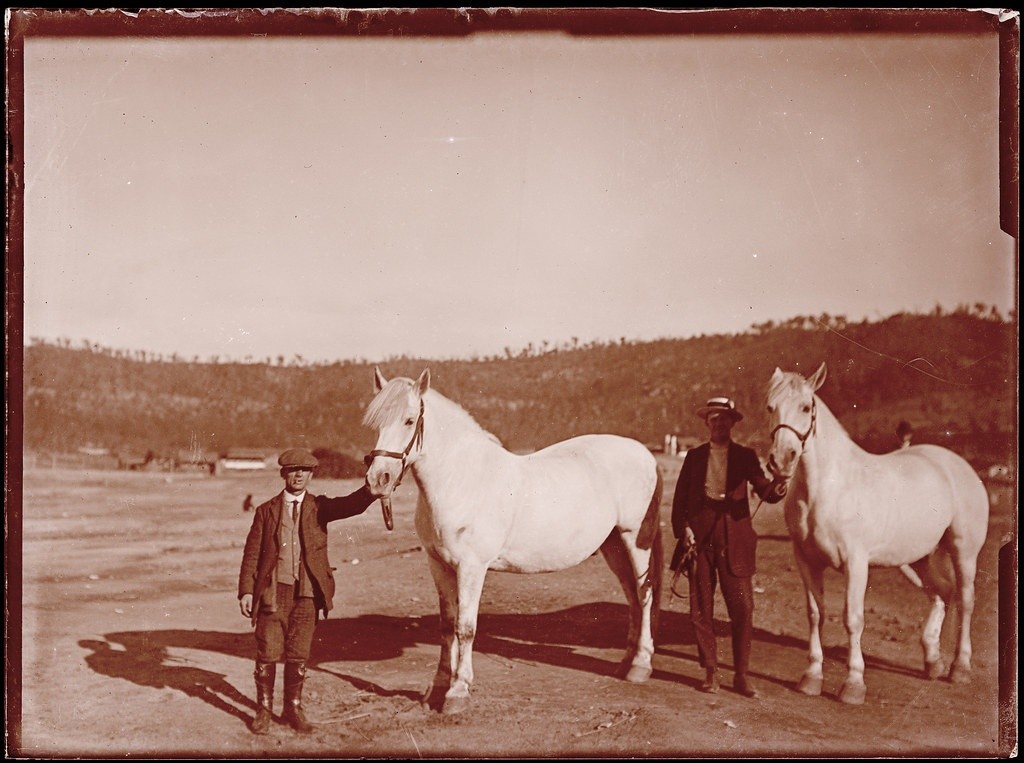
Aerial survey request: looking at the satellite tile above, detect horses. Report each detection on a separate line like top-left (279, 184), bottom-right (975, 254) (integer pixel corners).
top-left (762, 360), bottom-right (990, 705)
top-left (359, 363), bottom-right (666, 716)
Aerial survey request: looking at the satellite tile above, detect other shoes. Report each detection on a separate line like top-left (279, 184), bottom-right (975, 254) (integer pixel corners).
top-left (732, 676), bottom-right (759, 697)
top-left (703, 672), bottom-right (720, 693)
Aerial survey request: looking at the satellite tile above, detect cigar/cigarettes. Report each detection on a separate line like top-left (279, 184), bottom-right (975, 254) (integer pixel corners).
top-left (295, 480), bottom-right (298, 484)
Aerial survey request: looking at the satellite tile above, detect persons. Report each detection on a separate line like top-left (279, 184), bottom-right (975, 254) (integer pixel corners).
top-left (668, 396), bottom-right (788, 699)
top-left (236, 448), bottom-right (379, 736)
top-left (891, 420), bottom-right (918, 452)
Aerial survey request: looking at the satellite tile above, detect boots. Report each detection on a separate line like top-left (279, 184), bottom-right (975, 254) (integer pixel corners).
top-left (250, 659), bottom-right (276, 734)
top-left (281, 658), bottom-right (318, 734)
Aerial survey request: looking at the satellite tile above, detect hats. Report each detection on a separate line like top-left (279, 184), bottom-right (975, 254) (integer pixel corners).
top-left (696, 398), bottom-right (744, 421)
top-left (278, 449), bottom-right (320, 470)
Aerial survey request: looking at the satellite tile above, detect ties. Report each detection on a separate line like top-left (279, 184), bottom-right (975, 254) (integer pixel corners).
top-left (291, 500), bottom-right (300, 524)
